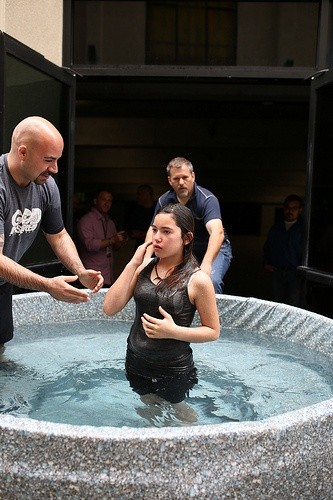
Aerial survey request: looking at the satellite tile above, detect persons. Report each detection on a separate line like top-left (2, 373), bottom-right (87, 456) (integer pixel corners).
top-left (78, 192), bottom-right (125, 288)
top-left (0, 116), bottom-right (103, 371)
top-left (145, 157), bottom-right (232, 294)
top-left (266, 195), bottom-right (305, 308)
top-left (102, 204), bottom-right (220, 423)
top-left (47, 189), bottom-right (83, 289)
top-left (130, 185), bottom-right (158, 259)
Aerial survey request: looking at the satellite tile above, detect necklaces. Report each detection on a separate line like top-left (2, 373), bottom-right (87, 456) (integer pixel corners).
top-left (100, 217), bottom-right (108, 239)
top-left (153, 263), bottom-right (163, 281)
